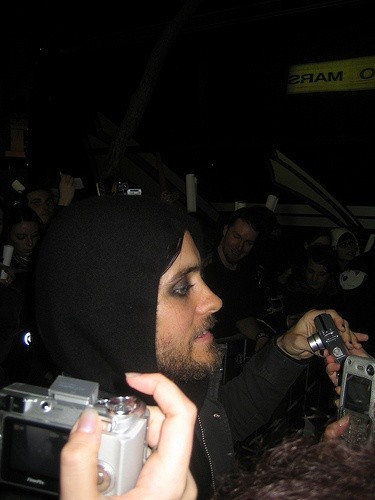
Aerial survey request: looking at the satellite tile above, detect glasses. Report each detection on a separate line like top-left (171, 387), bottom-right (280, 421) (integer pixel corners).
top-left (341, 240), bottom-right (355, 248)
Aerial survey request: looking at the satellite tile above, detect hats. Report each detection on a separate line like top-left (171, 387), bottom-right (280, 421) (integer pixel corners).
top-left (331, 228), bottom-right (359, 256)
top-left (311, 236), bottom-right (330, 244)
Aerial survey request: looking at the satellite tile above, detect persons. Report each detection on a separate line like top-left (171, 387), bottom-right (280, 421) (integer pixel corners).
top-left (212, 416), bottom-right (375, 500)
top-left (60, 371), bottom-right (199, 500)
top-left (27, 195), bottom-right (369, 486)
top-left (0, 175), bottom-right (375, 408)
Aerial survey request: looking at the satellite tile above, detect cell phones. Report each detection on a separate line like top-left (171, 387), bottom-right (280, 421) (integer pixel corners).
top-left (338, 356), bottom-right (375, 450)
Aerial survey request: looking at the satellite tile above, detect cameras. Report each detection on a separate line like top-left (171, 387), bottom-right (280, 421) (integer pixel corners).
top-left (0, 375), bottom-right (152, 500)
top-left (307, 313), bottom-right (348, 363)
top-left (116, 181), bottom-right (141, 196)
